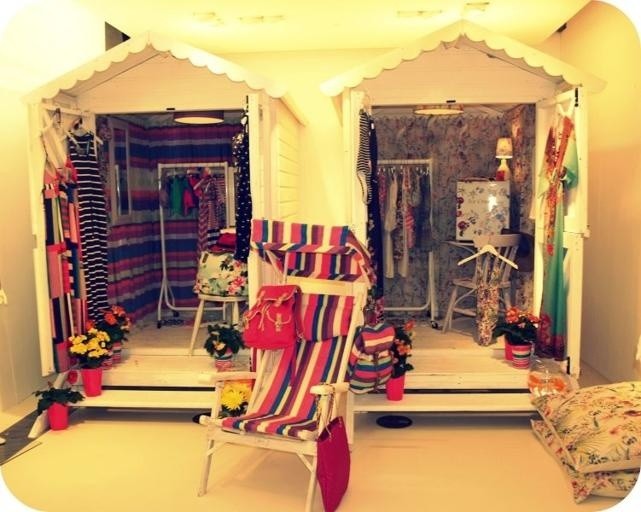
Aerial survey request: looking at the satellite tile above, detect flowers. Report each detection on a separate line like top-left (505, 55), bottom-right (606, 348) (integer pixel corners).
top-left (88, 328), bottom-right (112, 359)
top-left (492, 305), bottom-right (539, 345)
top-left (97, 304), bottom-right (131, 342)
top-left (68, 335), bottom-right (107, 367)
top-left (388, 320), bottom-right (417, 377)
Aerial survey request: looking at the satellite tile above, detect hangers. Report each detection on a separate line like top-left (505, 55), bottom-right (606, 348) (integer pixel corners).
top-left (551, 92), bottom-right (565, 126)
top-left (157, 162), bottom-right (228, 328)
top-left (458, 234), bottom-right (518, 270)
top-left (36, 113), bottom-right (78, 144)
top-left (57, 119), bottom-right (103, 146)
top-left (378, 157), bottom-right (439, 330)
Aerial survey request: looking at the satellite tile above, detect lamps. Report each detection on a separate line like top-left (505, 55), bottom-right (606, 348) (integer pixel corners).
top-left (413, 103), bottom-right (464, 116)
top-left (495, 138), bottom-right (513, 180)
top-left (173, 110), bottom-right (224, 125)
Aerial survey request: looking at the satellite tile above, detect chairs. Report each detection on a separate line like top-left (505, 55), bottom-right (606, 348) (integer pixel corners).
top-left (441, 233), bottom-right (521, 336)
top-left (193, 276), bottom-right (367, 512)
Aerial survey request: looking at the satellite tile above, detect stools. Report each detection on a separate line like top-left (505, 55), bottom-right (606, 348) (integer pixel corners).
top-left (188, 294), bottom-right (248, 357)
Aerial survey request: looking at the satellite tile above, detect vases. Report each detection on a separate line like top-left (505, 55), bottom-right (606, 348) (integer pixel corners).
top-left (385, 375), bottom-right (406, 401)
top-left (505, 337), bottom-right (514, 361)
top-left (80, 366), bottom-right (103, 397)
top-left (112, 341), bottom-right (122, 363)
top-left (101, 358), bottom-right (112, 370)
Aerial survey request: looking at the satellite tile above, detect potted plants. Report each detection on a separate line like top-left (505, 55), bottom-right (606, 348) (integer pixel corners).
top-left (205, 324), bottom-right (244, 370)
top-left (34, 387), bottom-right (85, 431)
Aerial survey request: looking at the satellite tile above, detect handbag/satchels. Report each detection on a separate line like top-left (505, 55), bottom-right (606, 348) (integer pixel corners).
top-left (315, 382), bottom-right (351, 512)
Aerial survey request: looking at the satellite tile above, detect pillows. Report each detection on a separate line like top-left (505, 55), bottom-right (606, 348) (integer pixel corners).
top-left (531, 419), bottom-right (640, 504)
top-left (532, 381), bottom-right (641, 472)
